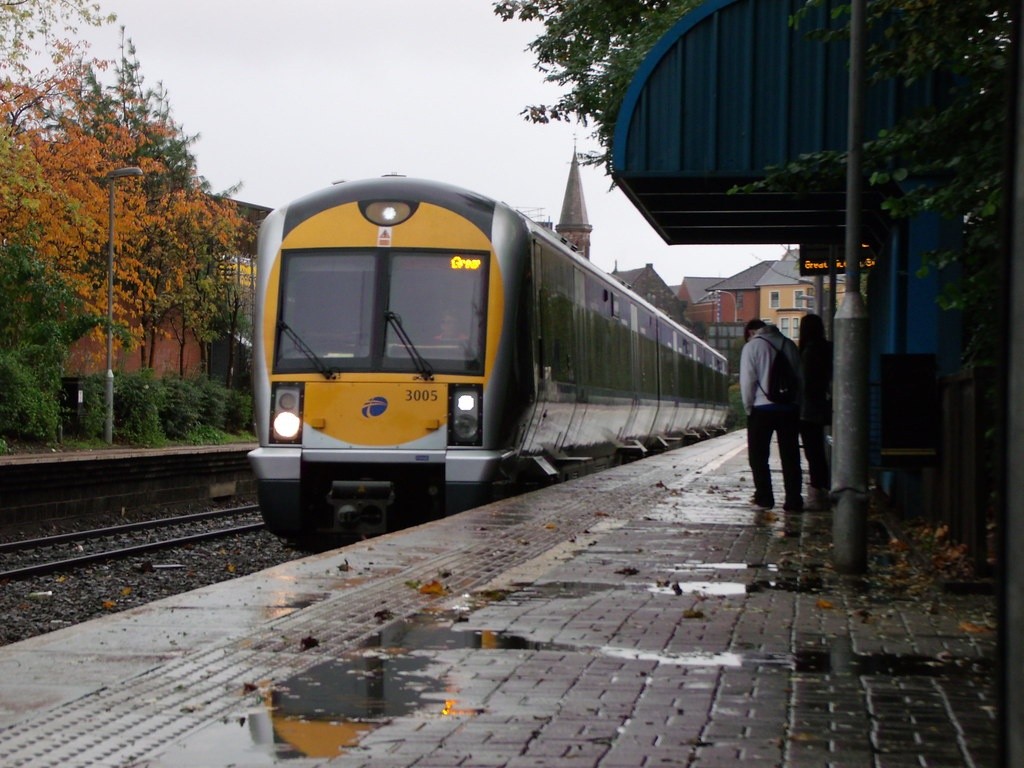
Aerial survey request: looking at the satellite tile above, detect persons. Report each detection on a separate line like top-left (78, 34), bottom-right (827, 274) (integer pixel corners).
top-left (798, 314), bottom-right (834, 512)
top-left (433, 313), bottom-right (468, 340)
top-left (740, 320), bottom-right (806, 512)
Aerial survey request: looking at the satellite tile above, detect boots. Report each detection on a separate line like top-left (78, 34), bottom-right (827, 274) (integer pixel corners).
top-left (802, 486), bottom-right (824, 510)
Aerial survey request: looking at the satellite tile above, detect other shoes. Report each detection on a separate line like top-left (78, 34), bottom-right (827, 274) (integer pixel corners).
top-left (751, 503), bottom-right (772, 510)
top-left (784, 501), bottom-right (803, 512)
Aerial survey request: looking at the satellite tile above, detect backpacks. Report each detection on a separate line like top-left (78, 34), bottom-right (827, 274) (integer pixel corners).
top-left (755, 336), bottom-right (799, 405)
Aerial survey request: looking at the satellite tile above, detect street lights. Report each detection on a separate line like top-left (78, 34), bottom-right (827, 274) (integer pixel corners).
top-left (104, 165), bottom-right (145, 448)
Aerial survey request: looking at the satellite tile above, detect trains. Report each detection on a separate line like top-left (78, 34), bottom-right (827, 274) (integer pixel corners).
top-left (245, 172), bottom-right (731, 548)
